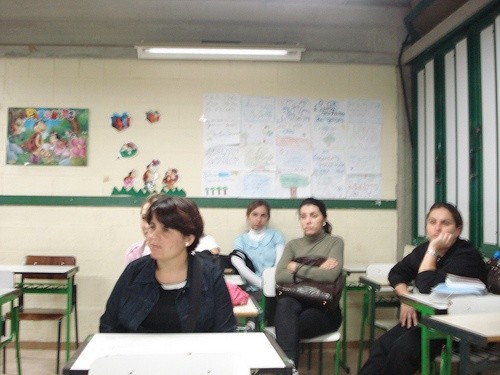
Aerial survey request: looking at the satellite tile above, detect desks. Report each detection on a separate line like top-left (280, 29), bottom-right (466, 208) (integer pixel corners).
top-left (234, 294), bottom-right (262, 317)
top-left (63, 331), bottom-right (294, 375)
top-left (421, 315), bottom-right (500, 375)
top-left (400, 295), bottom-right (458, 375)
top-left (339, 266), bottom-right (399, 375)
top-left (0, 264), bottom-right (80, 375)
top-left (225, 274), bottom-right (244, 286)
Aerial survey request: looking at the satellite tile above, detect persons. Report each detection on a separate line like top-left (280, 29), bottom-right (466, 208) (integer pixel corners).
top-left (99, 192), bottom-right (345, 375)
top-left (356, 202), bottom-right (488, 375)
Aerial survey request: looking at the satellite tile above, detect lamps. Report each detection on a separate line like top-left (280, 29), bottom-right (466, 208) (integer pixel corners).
top-left (134, 43), bottom-right (306, 61)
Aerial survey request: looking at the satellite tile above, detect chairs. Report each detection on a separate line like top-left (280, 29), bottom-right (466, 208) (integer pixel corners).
top-left (3, 256), bottom-right (79, 374)
top-left (261, 268), bottom-right (341, 375)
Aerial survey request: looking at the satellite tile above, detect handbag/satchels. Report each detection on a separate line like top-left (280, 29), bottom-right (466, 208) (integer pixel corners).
top-left (275, 255), bottom-right (346, 307)
top-left (484, 257), bottom-right (500, 295)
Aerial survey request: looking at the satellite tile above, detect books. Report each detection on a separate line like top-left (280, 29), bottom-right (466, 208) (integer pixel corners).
top-left (428, 273), bottom-right (486, 305)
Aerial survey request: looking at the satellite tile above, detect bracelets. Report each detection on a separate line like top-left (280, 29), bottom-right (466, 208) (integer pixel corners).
top-left (426, 248), bottom-right (441, 261)
